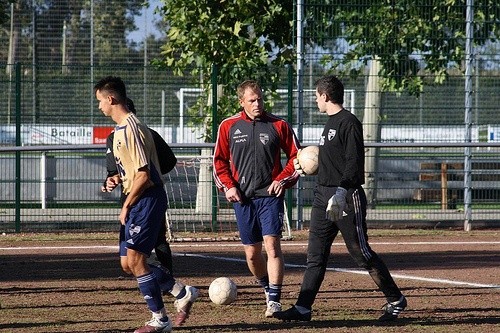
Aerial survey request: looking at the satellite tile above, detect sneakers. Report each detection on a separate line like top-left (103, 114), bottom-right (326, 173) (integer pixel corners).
top-left (272, 305), bottom-right (311, 322)
top-left (266, 292), bottom-right (270, 304)
top-left (377, 296), bottom-right (407, 323)
top-left (265, 301), bottom-right (281, 317)
top-left (134, 316), bottom-right (171, 333)
top-left (174, 285), bottom-right (198, 325)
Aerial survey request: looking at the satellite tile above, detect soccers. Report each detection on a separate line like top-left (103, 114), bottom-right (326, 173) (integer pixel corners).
top-left (297, 144), bottom-right (322, 175)
top-left (208, 276), bottom-right (237, 305)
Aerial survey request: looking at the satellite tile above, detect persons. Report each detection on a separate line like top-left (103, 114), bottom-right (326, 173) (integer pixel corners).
top-left (273, 76), bottom-right (408, 322)
top-left (212, 80), bottom-right (303, 316)
top-left (93, 76), bottom-right (197, 333)
top-left (102, 99), bottom-right (176, 295)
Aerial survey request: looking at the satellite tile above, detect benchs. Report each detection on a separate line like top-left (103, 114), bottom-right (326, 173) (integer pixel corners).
top-left (413, 162), bottom-right (500, 209)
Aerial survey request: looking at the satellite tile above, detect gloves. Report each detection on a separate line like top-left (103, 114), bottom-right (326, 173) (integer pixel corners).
top-left (292, 149), bottom-right (306, 177)
top-left (326, 187), bottom-right (348, 222)
